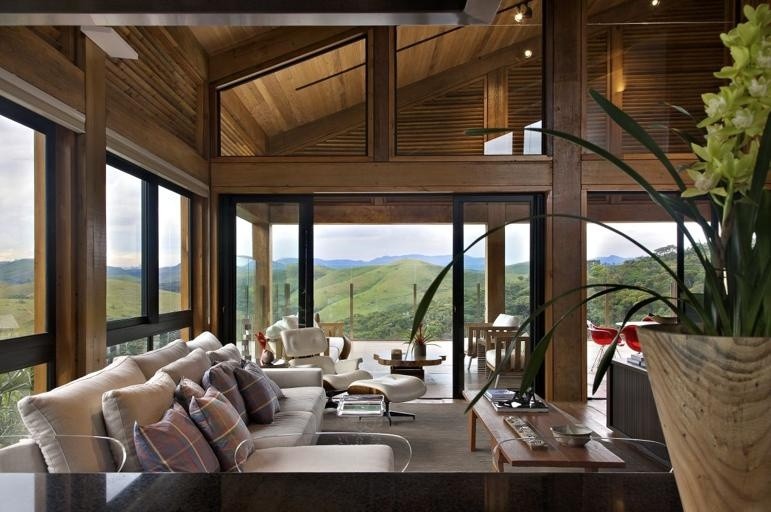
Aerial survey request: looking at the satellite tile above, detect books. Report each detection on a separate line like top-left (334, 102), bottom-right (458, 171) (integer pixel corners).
top-left (483, 389), bottom-right (549, 413)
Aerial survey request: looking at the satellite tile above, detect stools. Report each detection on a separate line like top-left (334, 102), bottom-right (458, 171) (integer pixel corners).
top-left (348, 372), bottom-right (427, 427)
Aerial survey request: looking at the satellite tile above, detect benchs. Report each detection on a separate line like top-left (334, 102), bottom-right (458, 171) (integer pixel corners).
top-left (464, 313), bottom-right (530, 390)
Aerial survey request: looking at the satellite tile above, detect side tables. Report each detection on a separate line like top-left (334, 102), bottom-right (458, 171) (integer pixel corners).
top-left (374, 352), bottom-right (446, 381)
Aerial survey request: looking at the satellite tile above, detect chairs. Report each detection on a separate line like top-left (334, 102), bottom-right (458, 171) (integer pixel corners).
top-left (585, 314), bottom-right (622, 374)
top-left (283, 326), bottom-right (373, 410)
top-left (621, 323), bottom-right (646, 356)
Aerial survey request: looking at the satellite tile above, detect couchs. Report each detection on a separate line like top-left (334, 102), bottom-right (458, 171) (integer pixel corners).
top-left (267, 313), bottom-right (349, 367)
top-left (0, 356), bottom-right (396, 472)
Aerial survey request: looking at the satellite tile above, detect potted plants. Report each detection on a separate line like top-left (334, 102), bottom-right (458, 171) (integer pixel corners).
top-left (403, 322), bottom-right (437, 361)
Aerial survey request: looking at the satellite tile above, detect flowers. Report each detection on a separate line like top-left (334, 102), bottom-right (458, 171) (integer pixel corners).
top-left (409, 4), bottom-right (771, 415)
top-left (254, 332), bottom-right (267, 350)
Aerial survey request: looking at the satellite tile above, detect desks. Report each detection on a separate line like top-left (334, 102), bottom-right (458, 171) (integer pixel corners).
top-left (607, 358), bottom-right (672, 473)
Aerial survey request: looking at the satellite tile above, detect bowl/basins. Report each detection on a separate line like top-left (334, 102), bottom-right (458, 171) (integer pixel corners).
top-left (550, 424), bottom-right (593, 447)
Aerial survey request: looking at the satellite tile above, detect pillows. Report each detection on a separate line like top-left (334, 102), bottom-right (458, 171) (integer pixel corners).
top-left (133, 400), bottom-right (220, 473)
top-left (186, 330), bottom-right (224, 353)
top-left (283, 316), bottom-right (301, 330)
top-left (174, 374), bottom-right (205, 408)
top-left (156, 348), bottom-right (213, 385)
top-left (189, 388), bottom-right (256, 472)
top-left (202, 342), bottom-right (285, 426)
top-left (16, 355), bottom-right (147, 473)
top-left (113, 338), bottom-right (191, 376)
top-left (101, 370), bottom-right (176, 473)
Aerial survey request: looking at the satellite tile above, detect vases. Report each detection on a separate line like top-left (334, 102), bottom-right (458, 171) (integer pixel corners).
top-left (634, 323), bottom-right (771, 512)
top-left (261, 350), bottom-right (274, 364)
